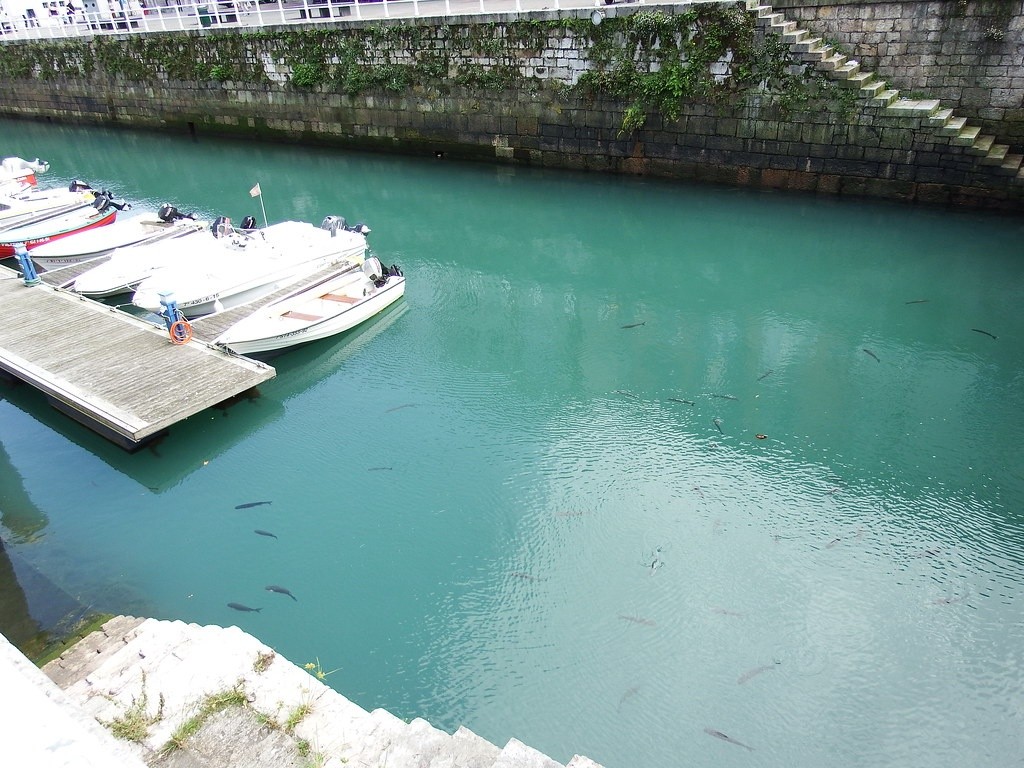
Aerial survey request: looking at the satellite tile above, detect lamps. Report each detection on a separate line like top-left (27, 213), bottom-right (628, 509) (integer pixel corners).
top-left (591, 10), bottom-right (606, 26)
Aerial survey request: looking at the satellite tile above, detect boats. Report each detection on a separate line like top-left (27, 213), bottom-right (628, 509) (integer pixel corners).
top-left (132, 216), bottom-right (371, 319)
top-left (28, 203), bottom-right (198, 271)
top-left (0, 156), bottom-right (132, 261)
top-left (75, 216), bottom-right (256, 299)
top-left (218, 256), bottom-right (406, 355)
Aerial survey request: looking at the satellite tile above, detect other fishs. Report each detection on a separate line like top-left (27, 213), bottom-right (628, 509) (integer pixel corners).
top-left (227, 501), bottom-right (298, 614)
top-left (507, 299), bottom-right (1020, 752)
top-left (384, 403), bottom-right (416, 413)
top-left (367, 467), bottom-right (393, 471)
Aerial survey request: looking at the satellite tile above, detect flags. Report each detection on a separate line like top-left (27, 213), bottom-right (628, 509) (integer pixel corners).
top-left (250, 184), bottom-right (261, 197)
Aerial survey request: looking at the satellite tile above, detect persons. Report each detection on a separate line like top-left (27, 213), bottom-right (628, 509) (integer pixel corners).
top-left (235, 0), bottom-right (252, 16)
top-left (48, 0), bottom-right (76, 25)
